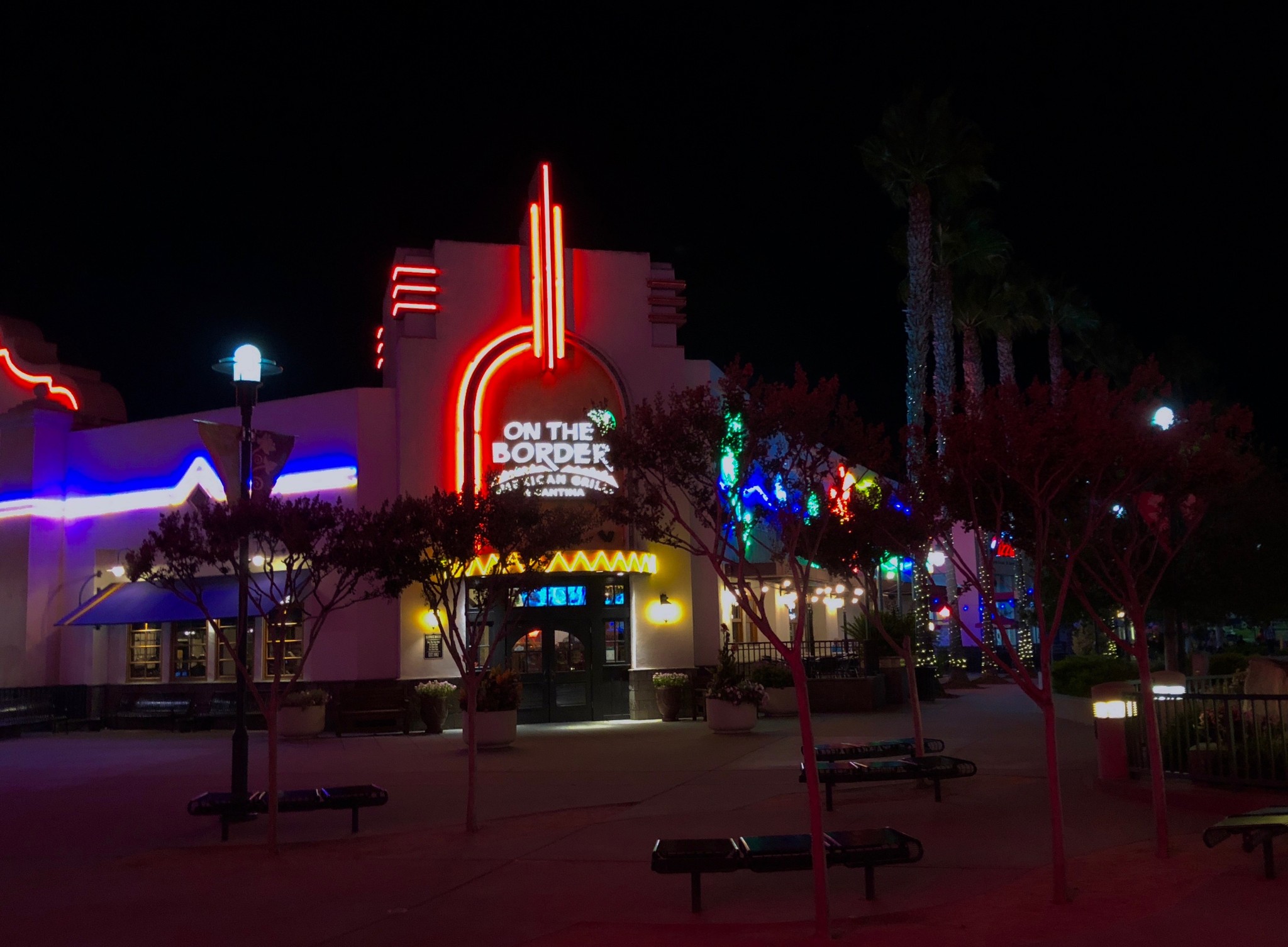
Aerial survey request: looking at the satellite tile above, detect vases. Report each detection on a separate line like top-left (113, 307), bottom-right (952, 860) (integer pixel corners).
top-left (706, 697), bottom-right (758, 733)
top-left (656, 686), bottom-right (680, 722)
top-left (420, 695), bottom-right (448, 732)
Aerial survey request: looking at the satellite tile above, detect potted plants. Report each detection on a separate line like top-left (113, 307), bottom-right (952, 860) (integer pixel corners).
top-left (278, 689), bottom-right (331, 740)
top-left (458, 664), bottom-right (523, 749)
top-left (754, 655), bottom-right (800, 717)
top-left (842, 612), bottom-right (919, 668)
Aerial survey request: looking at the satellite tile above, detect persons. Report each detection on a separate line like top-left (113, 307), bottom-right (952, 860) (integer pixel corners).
top-left (191, 656), bottom-right (205, 673)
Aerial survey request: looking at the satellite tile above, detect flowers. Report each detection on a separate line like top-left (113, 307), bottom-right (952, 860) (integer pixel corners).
top-left (704, 620), bottom-right (771, 706)
top-left (414, 680), bottom-right (456, 699)
top-left (653, 672), bottom-right (689, 687)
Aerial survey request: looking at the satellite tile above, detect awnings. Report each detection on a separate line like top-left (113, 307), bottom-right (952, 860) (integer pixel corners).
top-left (53, 568), bottom-right (314, 627)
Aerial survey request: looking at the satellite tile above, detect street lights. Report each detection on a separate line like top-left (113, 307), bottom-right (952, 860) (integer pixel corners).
top-left (215, 341), bottom-right (266, 795)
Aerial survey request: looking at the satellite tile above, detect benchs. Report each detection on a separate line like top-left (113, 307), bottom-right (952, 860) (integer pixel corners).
top-left (334, 679), bottom-right (411, 737)
top-left (1202, 803), bottom-right (1288, 880)
top-left (649, 828), bottom-right (925, 913)
top-left (188, 785), bottom-right (389, 841)
top-left (800, 756), bottom-right (978, 812)
top-left (106, 692), bottom-right (193, 732)
top-left (0, 685), bottom-right (68, 735)
top-left (801, 737), bottom-right (944, 787)
top-left (197, 690), bottom-right (270, 731)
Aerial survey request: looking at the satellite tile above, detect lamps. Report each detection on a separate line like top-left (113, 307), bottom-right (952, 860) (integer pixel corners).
top-left (1093, 671), bottom-right (1187, 719)
